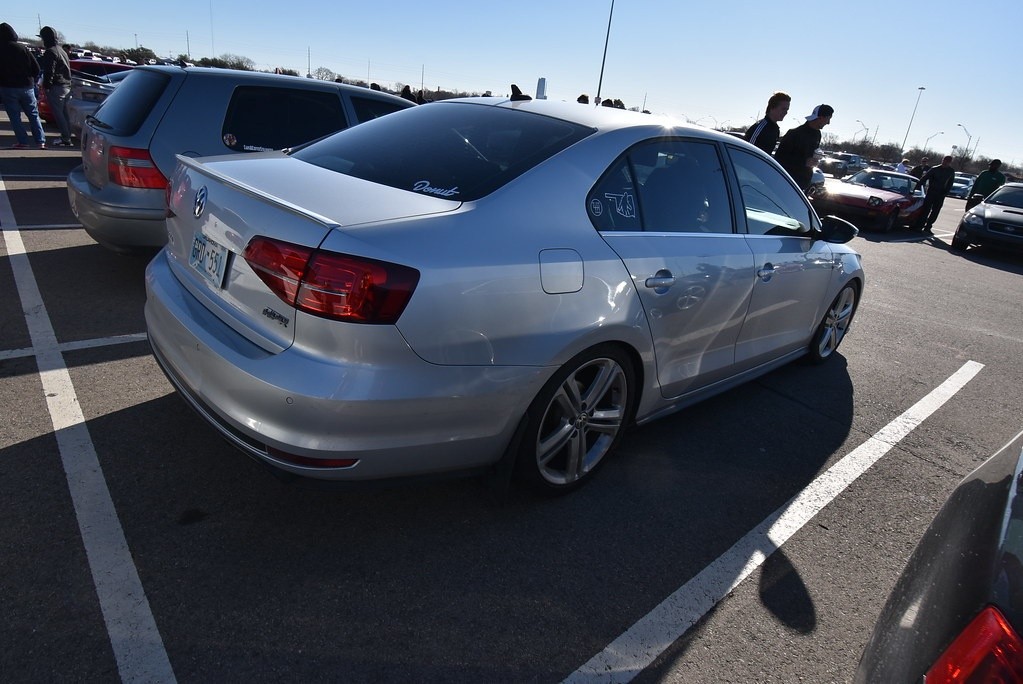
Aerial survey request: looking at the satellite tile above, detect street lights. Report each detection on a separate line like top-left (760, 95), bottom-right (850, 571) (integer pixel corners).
top-left (900, 87), bottom-right (925, 154)
top-left (924, 132), bottom-right (944, 151)
top-left (857, 120), bottom-right (868, 140)
top-left (957, 124), bottom-right (972, 171)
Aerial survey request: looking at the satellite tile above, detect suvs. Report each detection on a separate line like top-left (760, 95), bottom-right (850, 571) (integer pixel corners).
top-left (67, 62), bottom-right (484, 259)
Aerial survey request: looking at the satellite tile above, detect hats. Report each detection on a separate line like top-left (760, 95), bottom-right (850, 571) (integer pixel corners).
top-left (988, 159), bottom-right (1002, 165)
top-left (805, 104), bottom-right (834, 121)
top-left (36, 26), bottom-right (58, 39)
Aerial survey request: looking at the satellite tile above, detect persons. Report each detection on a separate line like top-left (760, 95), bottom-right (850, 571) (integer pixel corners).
top-left (62, 44), bottom-right (80, 60)
top-left (577, 95), bottom-right (588, 104)
top-left (773, 104), bottom-right (834, 191)
top-left (744, 93), bottom-right (791, 156)
top-left (895, 159), bottom-right (910, 174)
top-left (601, 99), bottom-right (614, 107)
top-left (335, 79), bottom-right (343, 83)
top-left (400, 85), bottom-right (417, 103)
top-left (965, 159), bottom-right (1007, 212)
top-left (913, 156), bottom-right (956, 234)
top-left (481, 94), bottom-right (492, 97)
top-left (371, 83), bottom-right (381, 91)
top-left (30, 47), bottom-right (42, 60)
top-left (110, 52), bottom-right (151, 66)
top-left (0, 23), bottom-right (49, 149)
top-left (908, 157), bottom-right (932, 187)
top-left (36, 26), bottom-right (75, 147)
top-left (417, 90), bottom-right (427, 105)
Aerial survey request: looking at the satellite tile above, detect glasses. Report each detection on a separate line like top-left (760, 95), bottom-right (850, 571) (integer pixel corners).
top-left (922, 160), bottom-right (927, 163)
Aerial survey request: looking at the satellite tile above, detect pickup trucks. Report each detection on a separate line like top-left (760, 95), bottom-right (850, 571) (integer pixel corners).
top-left (818, 152), bottom-right (868, 179)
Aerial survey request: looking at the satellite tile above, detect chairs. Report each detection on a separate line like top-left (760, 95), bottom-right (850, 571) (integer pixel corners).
top-left (637, 167), bottom-right (700, 233)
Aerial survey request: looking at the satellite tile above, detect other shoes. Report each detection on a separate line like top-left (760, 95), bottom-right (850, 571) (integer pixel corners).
top-left (35, 143), bottom-right (48, 150)
top-left (923, 228), bottom-right (934, 235)
top-left (12, 142), bottom-right (32, 150)
top-left (51, 137), bottom-right (74, 146)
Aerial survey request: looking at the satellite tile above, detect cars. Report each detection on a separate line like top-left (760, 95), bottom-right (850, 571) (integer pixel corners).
top-left (950, 176), bottom-right (973, 198)
top-left (814, 148), bottom-right (832, 160)
top-left (34, 59), bottom-right (134, 131)
top-left (71, 48), bottom-right (137, 65)
top-left (869, 161), bottom-right (913, 173)
top-left (807, 168), bottom-right (926, 232)
top-left (951, 182), bottom-right (1023, 254)
top-left (853, 433), bottom-right (1023, 684)
top-left (144, 99), bottom-right (865, 502)
